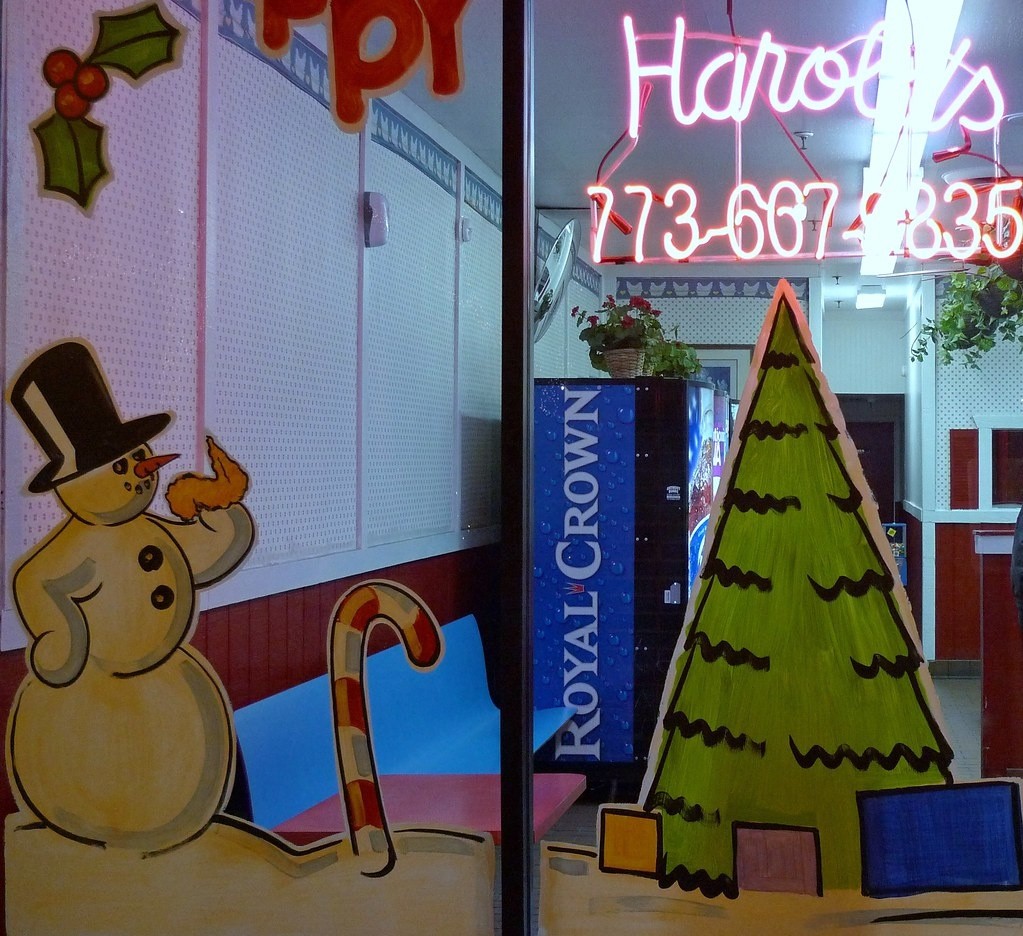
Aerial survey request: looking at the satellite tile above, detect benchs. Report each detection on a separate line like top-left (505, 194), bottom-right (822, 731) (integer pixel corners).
top-left (233, 613), bottom-right (577, 832)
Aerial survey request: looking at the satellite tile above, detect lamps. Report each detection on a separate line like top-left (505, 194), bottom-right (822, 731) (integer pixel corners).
top-left (856, 285), bottom-right (887, 309)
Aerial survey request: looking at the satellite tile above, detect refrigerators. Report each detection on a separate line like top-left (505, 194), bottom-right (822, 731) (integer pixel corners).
top-left (535, 378), bottom-right (741, 794)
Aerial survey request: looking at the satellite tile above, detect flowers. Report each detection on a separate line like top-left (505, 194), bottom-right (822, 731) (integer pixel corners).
top-left (571, 294), bottom-right (662, 372)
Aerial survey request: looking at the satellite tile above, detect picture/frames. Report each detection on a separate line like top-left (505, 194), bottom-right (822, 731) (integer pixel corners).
top-left (684, 344), bottom-right (755, 404)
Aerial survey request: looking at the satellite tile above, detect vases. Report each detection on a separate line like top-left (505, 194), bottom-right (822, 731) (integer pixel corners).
top-left (603, 349), bottom-right (646, 379)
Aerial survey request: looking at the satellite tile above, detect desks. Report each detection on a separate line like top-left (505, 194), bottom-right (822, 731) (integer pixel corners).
top-left (269, 775), bottom-right (587, 844)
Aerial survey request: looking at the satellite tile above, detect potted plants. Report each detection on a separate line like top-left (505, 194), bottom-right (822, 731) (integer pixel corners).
top-left (655, 325), bottom-right (702, 379)
top-left (642, 337), bottom-right (661, 376)
